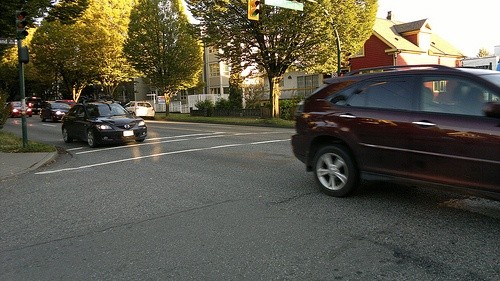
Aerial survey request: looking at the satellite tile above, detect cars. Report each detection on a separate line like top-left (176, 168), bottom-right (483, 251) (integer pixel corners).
top-left (61, 99), bottom-right (148, 149)
top-left (122, 100), bottom-right (155, 120)
top-left (39, 102), bottom-right (76, 123)
top-left (5, 95), bottom-right (80, 119)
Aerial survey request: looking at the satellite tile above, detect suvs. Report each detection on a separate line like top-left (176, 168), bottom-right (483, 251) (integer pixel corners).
top-left (291, 63), bottom-right (500, 202)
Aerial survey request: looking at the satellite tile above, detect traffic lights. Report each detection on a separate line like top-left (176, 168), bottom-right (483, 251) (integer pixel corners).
top-left (248, 0), bottom-right (261, 21)
top-left (16, 9), bottom-right (28, 39)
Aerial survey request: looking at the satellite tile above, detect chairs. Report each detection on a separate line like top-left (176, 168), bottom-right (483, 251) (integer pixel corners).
top-left (421, 87), bottom-right (441, 111)
